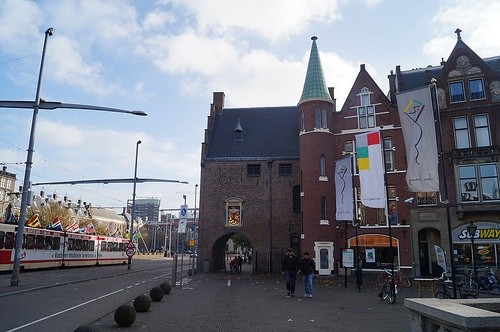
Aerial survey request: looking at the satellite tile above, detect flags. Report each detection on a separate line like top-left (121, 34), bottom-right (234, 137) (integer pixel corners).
top-left (64, 221), bottom-right (96, 234)
top-left (2, 203), bottom-right (17, 224)
top-left (109, 228), bottom-right (142, 239)
top-left (25, 213), bottom-right (42, 228)
top-left (47, 217), bottom-right (63, 231)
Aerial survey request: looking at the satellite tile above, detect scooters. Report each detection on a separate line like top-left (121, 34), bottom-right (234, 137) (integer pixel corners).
top-left (475, 268), bottom-right (500, 295)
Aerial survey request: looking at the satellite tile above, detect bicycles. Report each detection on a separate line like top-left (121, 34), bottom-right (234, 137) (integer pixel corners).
top-left (378, 265), bottom-right (411, 288)
top-left (379, 274), bottom-right (397, 304)
top-left (460, 271), bottom-right (478, 290)
top-left (434, 272), bottom-right (477, 299)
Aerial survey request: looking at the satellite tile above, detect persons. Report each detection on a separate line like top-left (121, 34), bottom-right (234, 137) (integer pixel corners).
top-left (229, 256), bottom-right (243, 273)
top-left (281, 248), bottom-right (317, 298)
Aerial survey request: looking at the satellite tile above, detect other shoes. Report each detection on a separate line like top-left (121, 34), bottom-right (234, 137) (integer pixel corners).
top-left (285, 291), bottom-right (296, 298)
top-left (303, 293), bottom-right (308, 297)
top-left (308, 294), bottom-right (313, 298)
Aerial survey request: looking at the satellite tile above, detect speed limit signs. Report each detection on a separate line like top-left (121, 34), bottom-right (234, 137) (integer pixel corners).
top-left (126, 248), bottom-right (134, 257)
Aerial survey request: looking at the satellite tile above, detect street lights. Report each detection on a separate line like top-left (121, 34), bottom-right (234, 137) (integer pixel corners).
top-left (466, 221), bottom-right (477, 282)
top-left (33, 138), bottom-right (189, 271)
top-left (193, 184), bottom-right (198, 268)
top-left (0, 26), bottom-right (147, 290)
